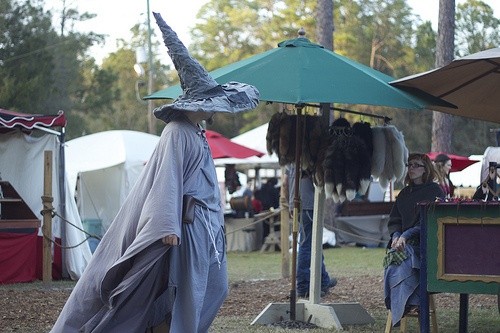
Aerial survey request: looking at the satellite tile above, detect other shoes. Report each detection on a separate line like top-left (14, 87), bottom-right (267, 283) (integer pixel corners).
top-left (296, 290), bottom-right (325, 298)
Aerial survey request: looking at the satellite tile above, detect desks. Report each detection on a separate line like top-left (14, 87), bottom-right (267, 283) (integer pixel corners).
top-left (415, 202), bottom-right (500, 333)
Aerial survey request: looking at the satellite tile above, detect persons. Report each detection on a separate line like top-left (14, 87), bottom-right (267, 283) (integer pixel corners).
top-left (433, 154), bottom-right (455, 199)
top-left (47, 10), bottom-right (260, 333)
top-left (384, 154), bottom-right (447, 328)
top-left (243, 161), bottom-right (336, 298)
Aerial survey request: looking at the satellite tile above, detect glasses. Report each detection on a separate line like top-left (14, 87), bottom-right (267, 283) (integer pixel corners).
top-left (405, 162), bottom-right (425, 168)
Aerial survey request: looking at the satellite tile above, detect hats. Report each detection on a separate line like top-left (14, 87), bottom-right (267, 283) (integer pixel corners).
top-left (152, 11), bottom-right (260, 124)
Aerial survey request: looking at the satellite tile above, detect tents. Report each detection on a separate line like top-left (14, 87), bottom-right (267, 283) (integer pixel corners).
top-left (0, 109), bottom-right (281, 282)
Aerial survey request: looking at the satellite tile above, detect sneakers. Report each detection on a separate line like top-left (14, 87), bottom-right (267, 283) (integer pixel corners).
top-left (321, 279), bottom-right (337, 291)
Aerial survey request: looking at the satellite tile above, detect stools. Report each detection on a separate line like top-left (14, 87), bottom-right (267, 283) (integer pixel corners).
top-left (385, 295), bottom-right (438, 333)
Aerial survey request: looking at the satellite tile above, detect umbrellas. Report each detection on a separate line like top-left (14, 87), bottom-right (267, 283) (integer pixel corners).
top-left (388, 46), bottom-right (500, 126)
top-left (143, 29), bottom-right (458, 321)
top-left (204, 128), bottom-right (264, 159)
top-left (426, 150), bottom-right (478, 173)
